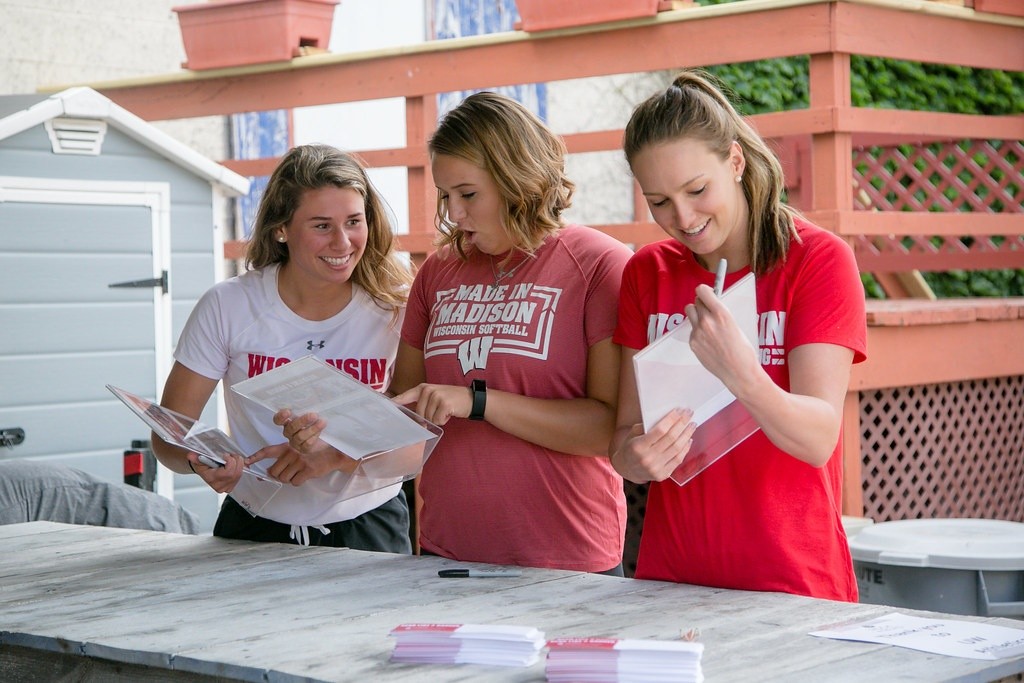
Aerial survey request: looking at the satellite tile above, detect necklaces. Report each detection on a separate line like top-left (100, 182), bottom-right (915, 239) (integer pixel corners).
top-left (490, 233), bottom-right (550, 289)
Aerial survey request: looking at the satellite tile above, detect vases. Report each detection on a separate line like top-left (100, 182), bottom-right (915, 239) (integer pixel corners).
top-left (512, 0), bottom-right (672, 32)
top-left (172, 0), bottom-right (341, 72)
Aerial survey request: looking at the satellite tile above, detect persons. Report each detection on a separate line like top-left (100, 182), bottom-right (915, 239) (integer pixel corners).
top-left (608, 69), bottom-right (867, 603)
top-left (273, 92), bottom-right (635, 578)
top-left (151, 143), bottom-right (413, 555)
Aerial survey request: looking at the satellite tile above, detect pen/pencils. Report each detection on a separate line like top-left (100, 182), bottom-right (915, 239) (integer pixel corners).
top-left (198, 455), bottom-right (219, 468)
top-left (714, 257), bottom-right (728, 297)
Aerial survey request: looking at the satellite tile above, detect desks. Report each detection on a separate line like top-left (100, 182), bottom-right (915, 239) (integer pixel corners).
top-left (0, 521), bottom-right (1024, 683)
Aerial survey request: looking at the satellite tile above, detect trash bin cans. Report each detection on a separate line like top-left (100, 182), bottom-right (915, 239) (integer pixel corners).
top-left (847, 519), bottom-right (1023, 622)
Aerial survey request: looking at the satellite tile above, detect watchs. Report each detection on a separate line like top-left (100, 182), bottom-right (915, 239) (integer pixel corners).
top-left (467, 378), bottom-right (487, 422)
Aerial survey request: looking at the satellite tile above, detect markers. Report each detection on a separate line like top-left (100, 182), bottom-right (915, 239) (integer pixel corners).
top-left (438, 569), bottom-right (522, 577)
